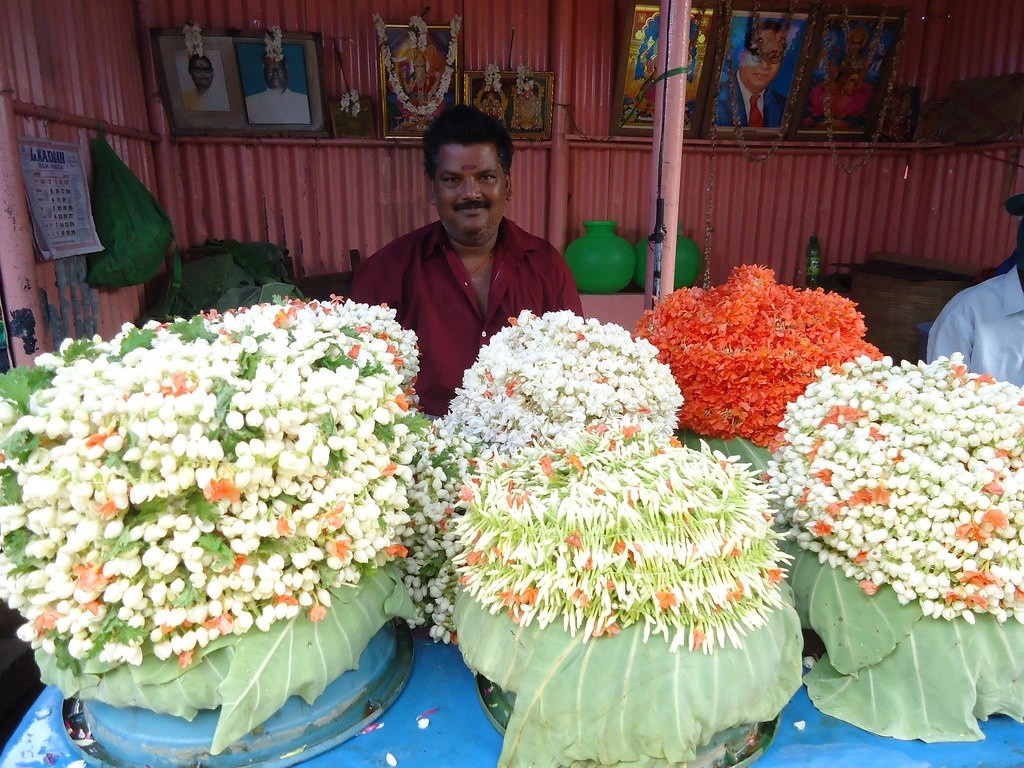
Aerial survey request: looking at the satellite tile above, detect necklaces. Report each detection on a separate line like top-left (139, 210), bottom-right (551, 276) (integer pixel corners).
top-left (469, 253), bottom-right (495, 274)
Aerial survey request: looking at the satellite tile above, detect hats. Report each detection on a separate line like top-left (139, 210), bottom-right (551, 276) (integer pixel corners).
top-left (1006, 193), bottom-right (1024, 215)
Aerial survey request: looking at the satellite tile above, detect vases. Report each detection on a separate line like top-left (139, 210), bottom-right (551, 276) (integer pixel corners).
top-left (564, 219), bottom-right (636, 294)
top-left (631, 221), bottom-right (702, 293)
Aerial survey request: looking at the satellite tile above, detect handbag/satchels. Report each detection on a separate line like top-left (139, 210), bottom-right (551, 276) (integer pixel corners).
top-left (83, 133), bottom-right (175, 287)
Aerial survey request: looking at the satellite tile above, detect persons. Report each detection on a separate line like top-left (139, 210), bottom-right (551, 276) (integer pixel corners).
top-left (926, 194), bottom-right (1024, 385)
top-left (184, 55), bottom-right (226, 111)
top-left (351, 105), bottom-right (586, 416)
top-left (246, 52), bottom-right (310, 123)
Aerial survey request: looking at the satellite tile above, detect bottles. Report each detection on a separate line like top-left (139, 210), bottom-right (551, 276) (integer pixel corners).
top-left (806, 235), bottom-right (822, 287)
top-left (636, 219), bottom-right (699, 293)
top-left (561, 219), bottom-right (636, 294)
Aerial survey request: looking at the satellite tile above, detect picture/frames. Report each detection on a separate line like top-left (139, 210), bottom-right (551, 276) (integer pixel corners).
top-left (377, 24), bottom-right (462, 140)
top-left (702, 7), bottom-right (818, 139)
top-left (463, 71), bottom-right (553, 141)
top-left (149, 25), bottom-right (332, 141)
top-left (609, 0), bottom-right (725, 137)
top-left (786, 8), bottom-right (910, 140)
